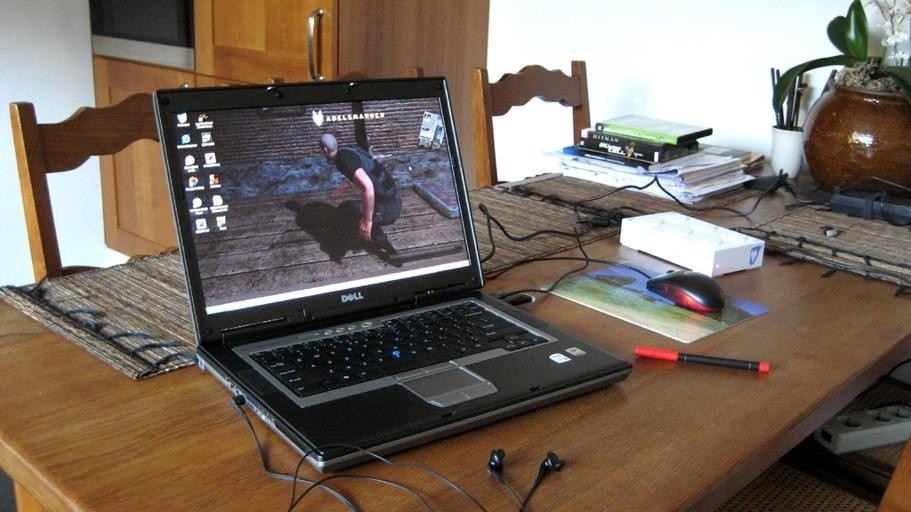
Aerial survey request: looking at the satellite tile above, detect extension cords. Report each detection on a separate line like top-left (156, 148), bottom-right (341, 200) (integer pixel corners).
top-left (815, 404), bottom-right (911, 455)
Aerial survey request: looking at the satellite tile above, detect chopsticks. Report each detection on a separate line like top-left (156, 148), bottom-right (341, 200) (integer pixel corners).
top-left (771, 67), bottom-right (838, 131)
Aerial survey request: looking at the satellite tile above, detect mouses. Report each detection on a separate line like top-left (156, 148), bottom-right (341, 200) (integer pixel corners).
top-left (647, 269), bottom-right (725, 314)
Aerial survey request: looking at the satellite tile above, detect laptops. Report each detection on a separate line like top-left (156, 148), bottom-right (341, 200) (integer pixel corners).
top-left (152, 75), bottom-right (632, 473)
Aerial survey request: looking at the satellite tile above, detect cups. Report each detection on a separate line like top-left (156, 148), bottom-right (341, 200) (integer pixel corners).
top-left (771, 125), bottom-right (805, 178)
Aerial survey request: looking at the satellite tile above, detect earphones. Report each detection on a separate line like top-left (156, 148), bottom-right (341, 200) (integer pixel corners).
top-left (535, 451), bottom-right (566, 483)
top-left (487, 449), bottom-right (506, 483)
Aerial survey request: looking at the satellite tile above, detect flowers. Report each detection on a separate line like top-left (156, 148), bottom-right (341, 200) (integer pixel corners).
top-left (769, 0), bottom-right (911, 115)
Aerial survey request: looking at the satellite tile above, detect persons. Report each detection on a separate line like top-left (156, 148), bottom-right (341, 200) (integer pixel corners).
top-left (319, 132), bottom-right (403, 253)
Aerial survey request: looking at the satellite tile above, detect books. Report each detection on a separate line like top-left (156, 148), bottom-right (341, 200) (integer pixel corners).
top-left (560, 114), bottom-right (767, 206)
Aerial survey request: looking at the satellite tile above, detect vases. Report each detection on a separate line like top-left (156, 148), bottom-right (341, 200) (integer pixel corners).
top-left (800, 84), bottom-right (911, 193)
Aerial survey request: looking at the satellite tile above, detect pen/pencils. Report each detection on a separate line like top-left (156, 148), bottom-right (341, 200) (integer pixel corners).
top-left (770, 66), bottom-right (804, 130)
top-left (632, 344), bottom-right (771, 378)
top-left (820, 69), bottom-right (838, 94)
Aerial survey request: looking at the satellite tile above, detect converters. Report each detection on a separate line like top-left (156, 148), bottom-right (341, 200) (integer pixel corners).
top-left (831, 185), bottom-right (911, 228)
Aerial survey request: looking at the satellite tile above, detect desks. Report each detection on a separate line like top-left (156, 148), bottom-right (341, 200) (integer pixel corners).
top-left (1, 173), bottom-right (910, 510)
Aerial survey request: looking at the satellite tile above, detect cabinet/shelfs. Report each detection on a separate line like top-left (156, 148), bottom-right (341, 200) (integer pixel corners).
top-left (193, 1), bottom-right (499, 192)
top-left (88, 56), bottom-right (285, 260)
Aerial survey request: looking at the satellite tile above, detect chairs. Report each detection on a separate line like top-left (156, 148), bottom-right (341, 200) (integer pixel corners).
top-left (796, 372), bottom-right (911, 500)
top-left (715, 426), bottom-right (911, 511)
top-left (9, 78), bottom-right (285, 283)
top-left (469, 55), bottom-right (593, 194)
